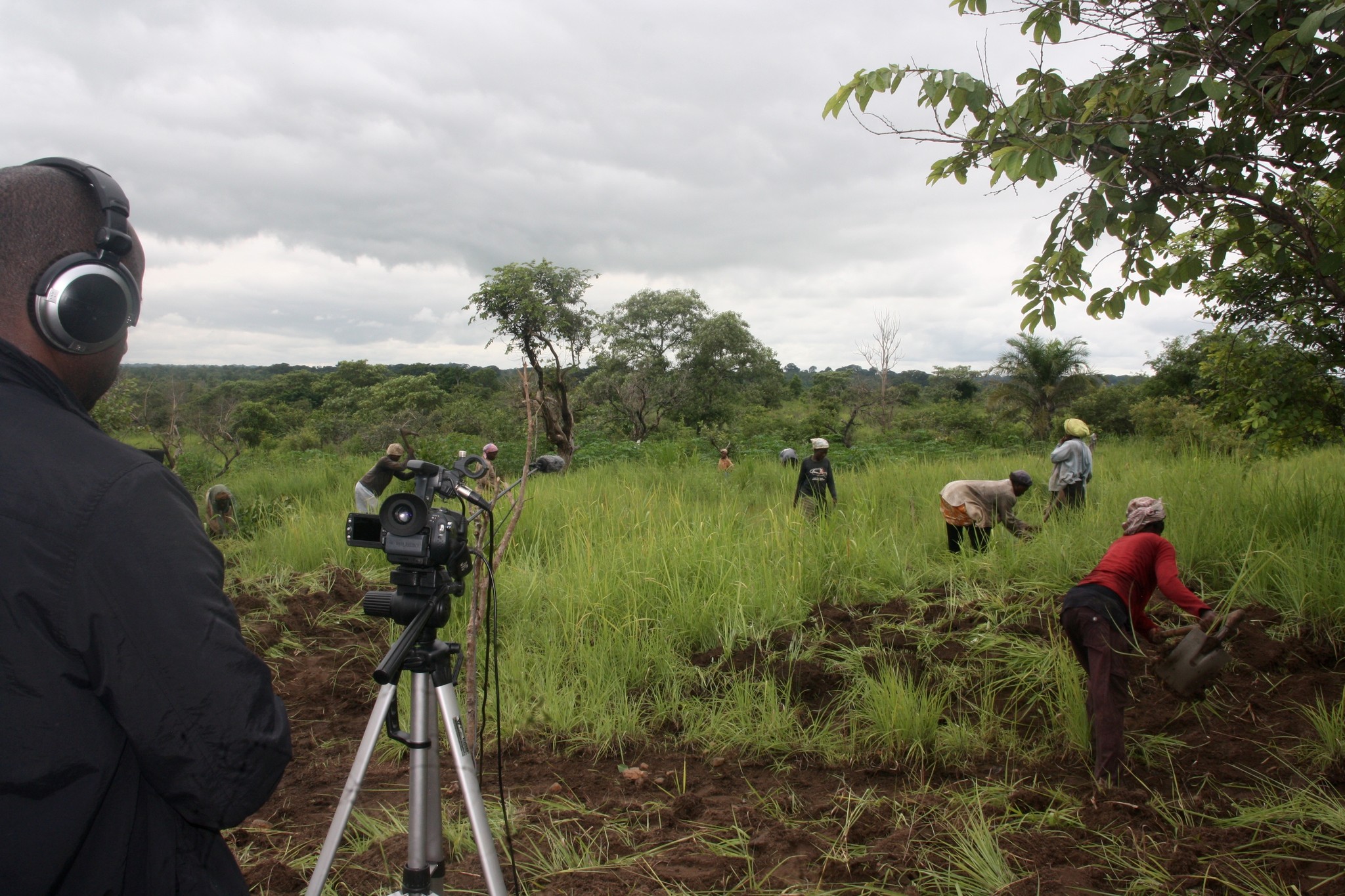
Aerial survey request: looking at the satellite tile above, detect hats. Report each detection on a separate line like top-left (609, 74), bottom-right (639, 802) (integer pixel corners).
top-left (386, 442), bottom-right (405, 457)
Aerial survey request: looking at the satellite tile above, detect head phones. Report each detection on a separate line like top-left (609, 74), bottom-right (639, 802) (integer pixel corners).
top-left (22, 156), bottom-right (141, 355)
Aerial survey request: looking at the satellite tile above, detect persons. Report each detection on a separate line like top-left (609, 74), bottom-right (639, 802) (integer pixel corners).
top-left (1089, 430), bottom-right (1098, 453)
top-left (354, 443), bottom-right (415, 516)
top-left (779, 447), bottom-right (798, 471)
top-left (1058, 495), bottom-right (1222, 805)
top-left (718, 448), bottom-right (734, 474)
top-left (793, 438), bottom-right (838, 526)
top-left (476, 443), bottom-right (502, 502)
top-left (939, 470), bottom-right (1043, 558)
top-left (1048, 417), bottom-right (1093, 520)
top-left (204, 483), bottom-right (234, 541)
top-left (0, 157), bottom-right (291, 896)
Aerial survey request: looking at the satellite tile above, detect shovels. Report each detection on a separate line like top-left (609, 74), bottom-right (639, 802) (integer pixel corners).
top-left (1151, 609), bottom-right (1246, 704)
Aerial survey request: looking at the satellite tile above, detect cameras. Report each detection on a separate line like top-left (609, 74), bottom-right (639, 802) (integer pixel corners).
top-left (346, 450), bottom-right (494, 591)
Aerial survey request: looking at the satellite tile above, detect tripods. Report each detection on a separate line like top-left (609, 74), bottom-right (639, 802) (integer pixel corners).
top-left (300, 589), bottom-right (510, 896)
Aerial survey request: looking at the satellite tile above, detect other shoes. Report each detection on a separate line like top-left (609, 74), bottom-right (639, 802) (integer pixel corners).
top-left (1123, 752), bottom-right (1139, 776)
